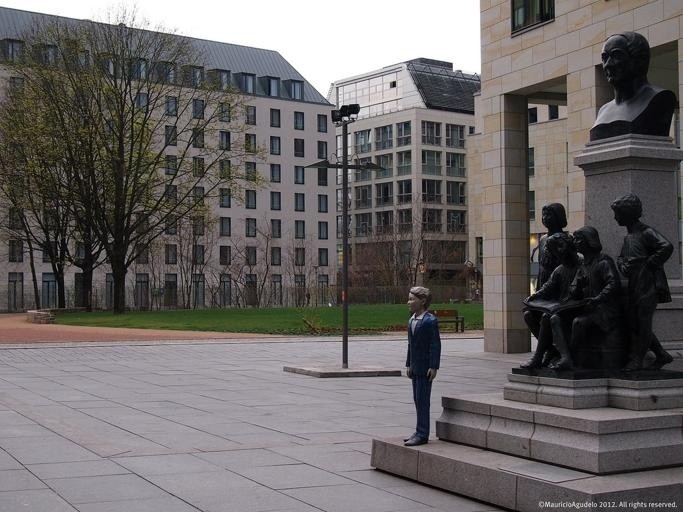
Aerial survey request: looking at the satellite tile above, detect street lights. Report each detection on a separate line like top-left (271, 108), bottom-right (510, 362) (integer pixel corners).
top-left (303, 103), bottom-right (385, 369)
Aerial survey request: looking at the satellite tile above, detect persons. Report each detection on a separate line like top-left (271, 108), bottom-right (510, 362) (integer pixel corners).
top-left (611, 191), bottom-right (674, 369)
top-left (521, 231), bottom-right (584, 368)
top-left (535, 200), bottom-right (568, 291)
top-left (403, 286), bottom-right (442, 448)
top-left (589, 31), bottom-right (668, 131)
top-left (450, 296), bottom-right (453, 304)
top-left (475, 287), bottom-right (480, 296)
top-left (545, 225), bottom-right (626, 371)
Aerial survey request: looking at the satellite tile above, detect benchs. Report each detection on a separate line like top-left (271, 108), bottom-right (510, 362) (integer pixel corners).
top-left (408, 309), bottom-right (465, 332)
